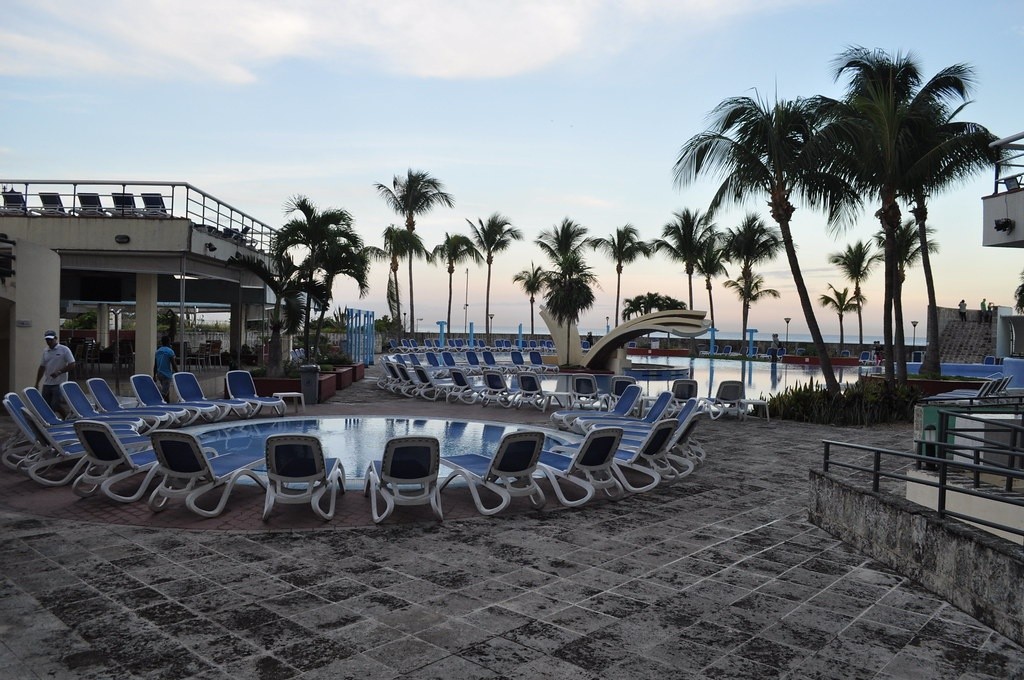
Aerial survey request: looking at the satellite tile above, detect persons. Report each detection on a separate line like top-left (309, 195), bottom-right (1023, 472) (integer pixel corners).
top-left (771, 333), bottom-right (780, 362)
top-left (981, 299), bottom-right (986, 322)
top-left (987, 302), bottom-right (994, 322)
top-left (958, 299), bottom-right (968, 323)
top-left (33, 330), bottom-right (76, 420)
top-left (153, 336), bottom-right (179, 404)
top-left (872, 341), bottom-right (884, 366)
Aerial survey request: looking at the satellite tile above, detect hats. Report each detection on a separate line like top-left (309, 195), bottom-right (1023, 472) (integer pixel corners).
top-left (44, 330), bottom-right (56, 339)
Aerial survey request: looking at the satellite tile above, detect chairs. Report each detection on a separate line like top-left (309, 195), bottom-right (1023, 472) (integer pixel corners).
top-left (927, 376), bottom-right (1015, 407)
top-left (77, 193), bottom-right (105, 216)
top-left (0, 191), bottom-right (32, 217)
top-left (0, 335), bottom-right (882, 524)
top-left (194, 223), bottom-right (250, 247)
top-left (139, 193), bottom-right (169, 218)
top-left (984, 356), bottom-right (1005, 365)
top-left (108, 193), bottom-right (140, 217)
top-left (37, 193), bottom-right (65, 216)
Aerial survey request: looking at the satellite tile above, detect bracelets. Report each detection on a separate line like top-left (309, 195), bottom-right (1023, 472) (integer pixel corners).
top-left (58, 369), bottom-right (63, 374)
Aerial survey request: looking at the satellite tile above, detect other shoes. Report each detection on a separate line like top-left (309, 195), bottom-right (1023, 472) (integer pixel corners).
top-left (63, 412), bottom-right (72, 421)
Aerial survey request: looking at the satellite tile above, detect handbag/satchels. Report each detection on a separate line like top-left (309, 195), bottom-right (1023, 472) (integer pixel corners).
top-left (778, 342), bottom-right (782, 348)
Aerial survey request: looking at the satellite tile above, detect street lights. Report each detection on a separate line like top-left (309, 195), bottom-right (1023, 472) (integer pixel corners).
top-left (110, 308), bottom-right (123, 395)
top-left (784, 318), bottom-right (791, 340)
top-left (911, 321), bottom-right (919, 345)
top-left (417, 318), bottom-right (423, 332)
top-left (489, 313), bottom-right (494, 345)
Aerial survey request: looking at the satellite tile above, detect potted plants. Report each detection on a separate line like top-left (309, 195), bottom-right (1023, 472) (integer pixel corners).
top-left (226, 195), bottom-right (372, 404)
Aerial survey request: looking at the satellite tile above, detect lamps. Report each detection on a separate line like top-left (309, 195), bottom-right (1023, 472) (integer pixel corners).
top-left (116, 235), bottom-right (130, 243)
top-left (994, 218), bottom-right (1016, 235)
top-left (206, 242), bottom-right (217, 252)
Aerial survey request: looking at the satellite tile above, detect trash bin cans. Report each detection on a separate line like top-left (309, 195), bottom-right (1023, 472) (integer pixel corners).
top-left (299, 365), bottom-right (321, 405)
